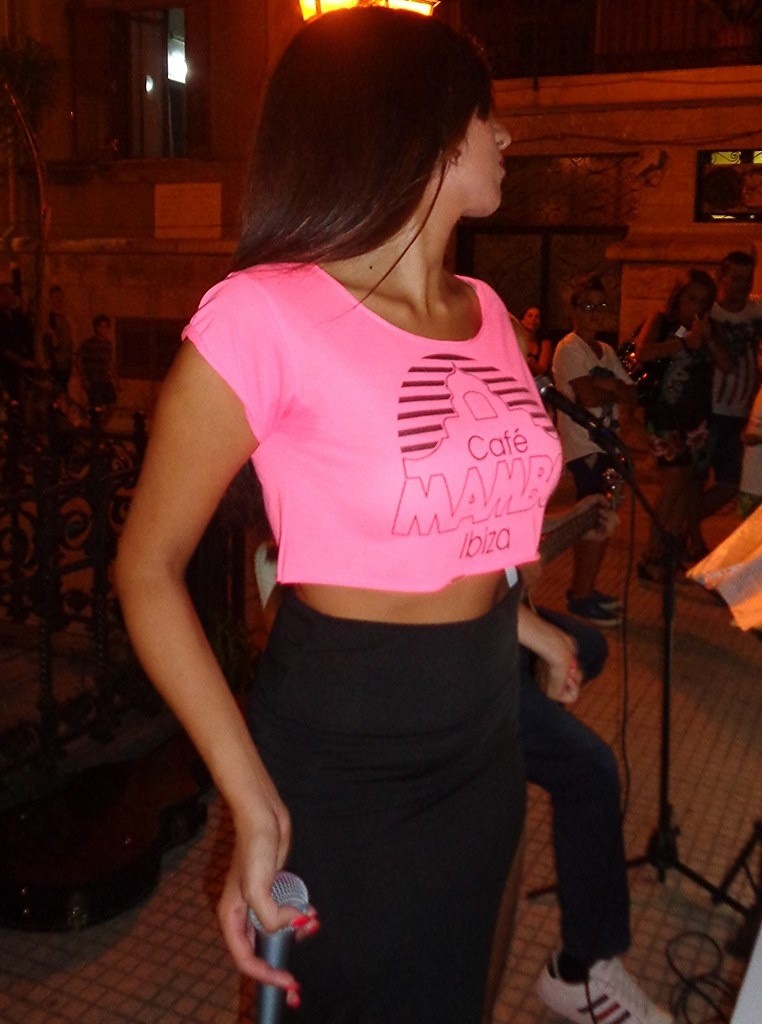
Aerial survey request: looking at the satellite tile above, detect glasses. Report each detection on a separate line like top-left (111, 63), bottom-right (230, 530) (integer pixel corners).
top-left (575, 300), bottom-right (610, 314)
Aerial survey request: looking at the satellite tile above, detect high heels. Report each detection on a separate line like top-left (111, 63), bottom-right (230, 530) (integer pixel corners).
top-left (638, 556), bottom-right (663, 589)
top-left (672, 562), bottom-right (689, 589)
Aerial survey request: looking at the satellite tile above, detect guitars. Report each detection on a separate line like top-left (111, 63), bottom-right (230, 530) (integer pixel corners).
top-left (538, 469), bottom-right (627, 565)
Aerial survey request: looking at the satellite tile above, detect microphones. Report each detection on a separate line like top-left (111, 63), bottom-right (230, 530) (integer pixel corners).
top-left (535, 375), bottom-right (623, 446)
top-left (249, 871), bottom-right (308, 1024)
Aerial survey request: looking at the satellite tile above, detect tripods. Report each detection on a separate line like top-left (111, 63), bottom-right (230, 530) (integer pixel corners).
top-left (524, 437), bottom-right (752, 927)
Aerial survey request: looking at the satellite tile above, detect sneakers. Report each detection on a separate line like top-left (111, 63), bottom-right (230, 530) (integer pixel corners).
top-left (568, 599), bottom-right (622, 628)
top-left (565, 589), bottom-right (617, 611)
top-left (533, 948), bottom-right (675, 1024)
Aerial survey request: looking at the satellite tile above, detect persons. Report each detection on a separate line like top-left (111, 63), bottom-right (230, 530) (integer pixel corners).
top-left (553, 280), bottom-right (637, 621)
top-left (637, 250), bottom-right (762, 584)
top-left (77, 314), bottom-right (117, 436)
top-left (42, 284), bottom-right (79, 408)
top-left (254, 309), bottom-right (674, 1024)
top-left (518, 305), bottom-right (550, 407)
top-left (116, 9), bottom-right (582, 1023)
